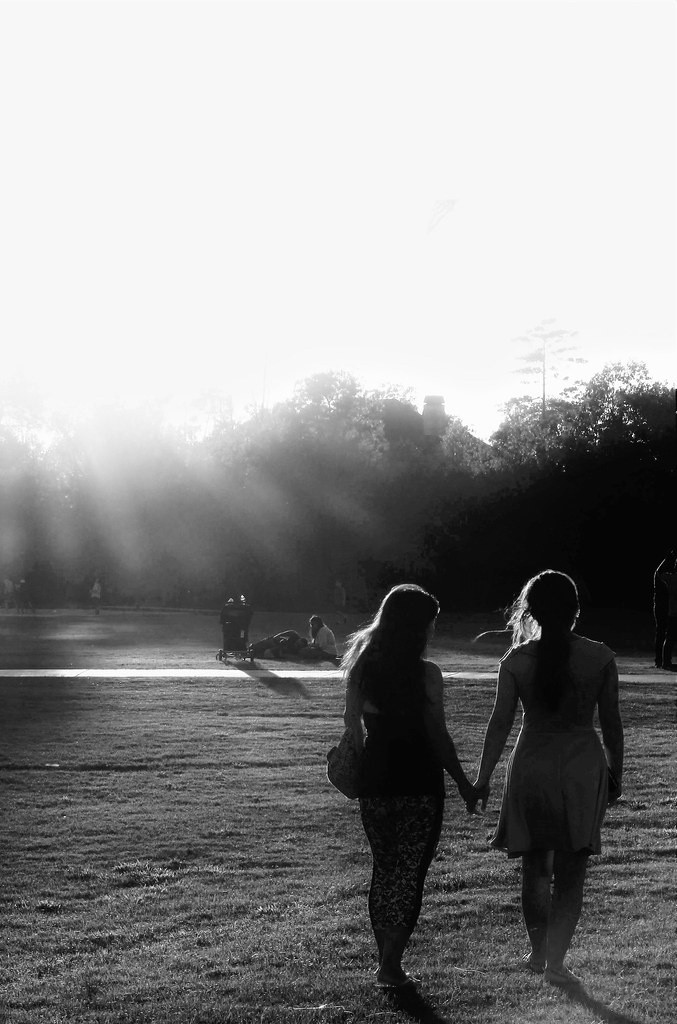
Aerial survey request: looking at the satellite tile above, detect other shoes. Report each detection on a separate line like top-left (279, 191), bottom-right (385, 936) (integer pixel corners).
top-left (545, 966), bottom-right (582, 984)
top-left (522, 951), bottom-right (544, 974)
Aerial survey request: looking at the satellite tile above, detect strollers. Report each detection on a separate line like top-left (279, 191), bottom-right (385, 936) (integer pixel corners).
top-left (216, 601), bottom-right (255, 663)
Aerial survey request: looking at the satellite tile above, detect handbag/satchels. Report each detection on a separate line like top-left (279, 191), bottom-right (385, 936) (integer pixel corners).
top-left (324, 646), bottom-right (378, 800)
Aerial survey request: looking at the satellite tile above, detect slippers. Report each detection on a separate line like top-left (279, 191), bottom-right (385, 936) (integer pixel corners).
top-left (376, 976), bottom-right (422, 991)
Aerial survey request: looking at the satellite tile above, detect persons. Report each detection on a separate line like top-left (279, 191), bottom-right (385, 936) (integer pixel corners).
top-left (465, 570), bottom-right (623, 985)
top-left (4, 574), bottom-right (102, 616)
top-left (273, 616), bottom-right (337, 661)
top-left (653, 550), bottom-right (677, 672)
top-left (325, 582), bottom-right (477, 989)
